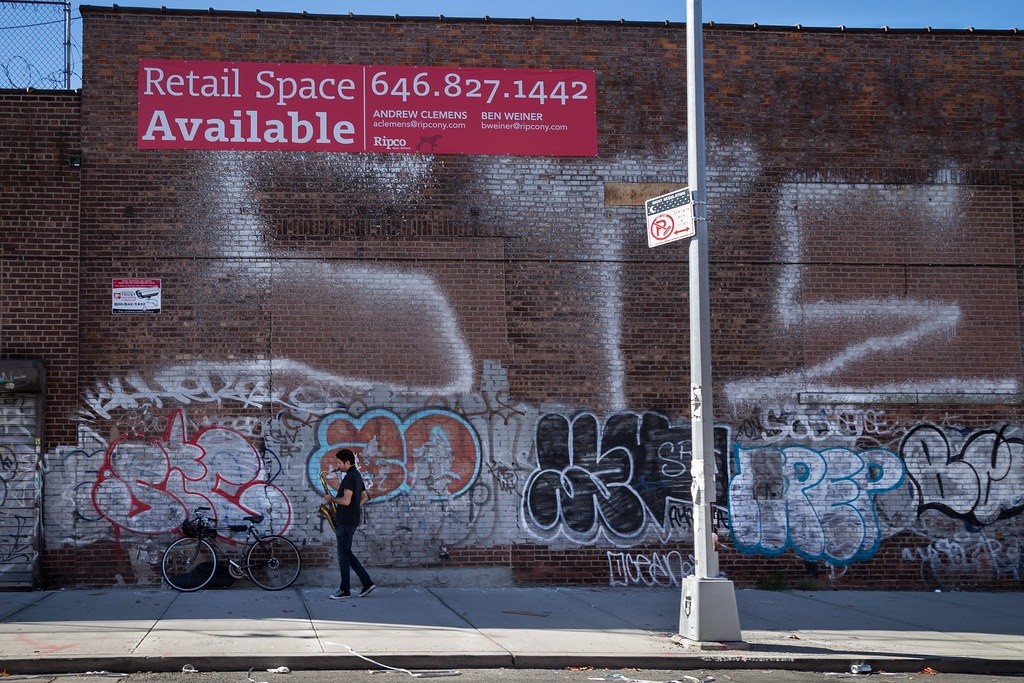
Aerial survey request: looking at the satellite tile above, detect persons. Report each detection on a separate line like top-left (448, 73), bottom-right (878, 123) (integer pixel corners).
top-left (323, 448), bottom-right (377, 598)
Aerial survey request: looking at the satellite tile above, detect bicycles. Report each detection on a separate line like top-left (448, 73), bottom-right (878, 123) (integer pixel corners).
top-left (162, 506), bottom-right (301, 591)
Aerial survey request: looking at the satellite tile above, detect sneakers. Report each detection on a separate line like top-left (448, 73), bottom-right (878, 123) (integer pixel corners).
top-left (329, 590), bottom-right (351, 599)
top-left (357, 582), bottom-right (375, 597)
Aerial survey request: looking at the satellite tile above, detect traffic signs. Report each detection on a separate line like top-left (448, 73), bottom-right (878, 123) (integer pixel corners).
top-left (645, 188), bottom-right (698, 249)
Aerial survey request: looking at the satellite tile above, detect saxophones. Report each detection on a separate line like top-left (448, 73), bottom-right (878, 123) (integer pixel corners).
top-left (318, 467), bottom-right (341, 533)
